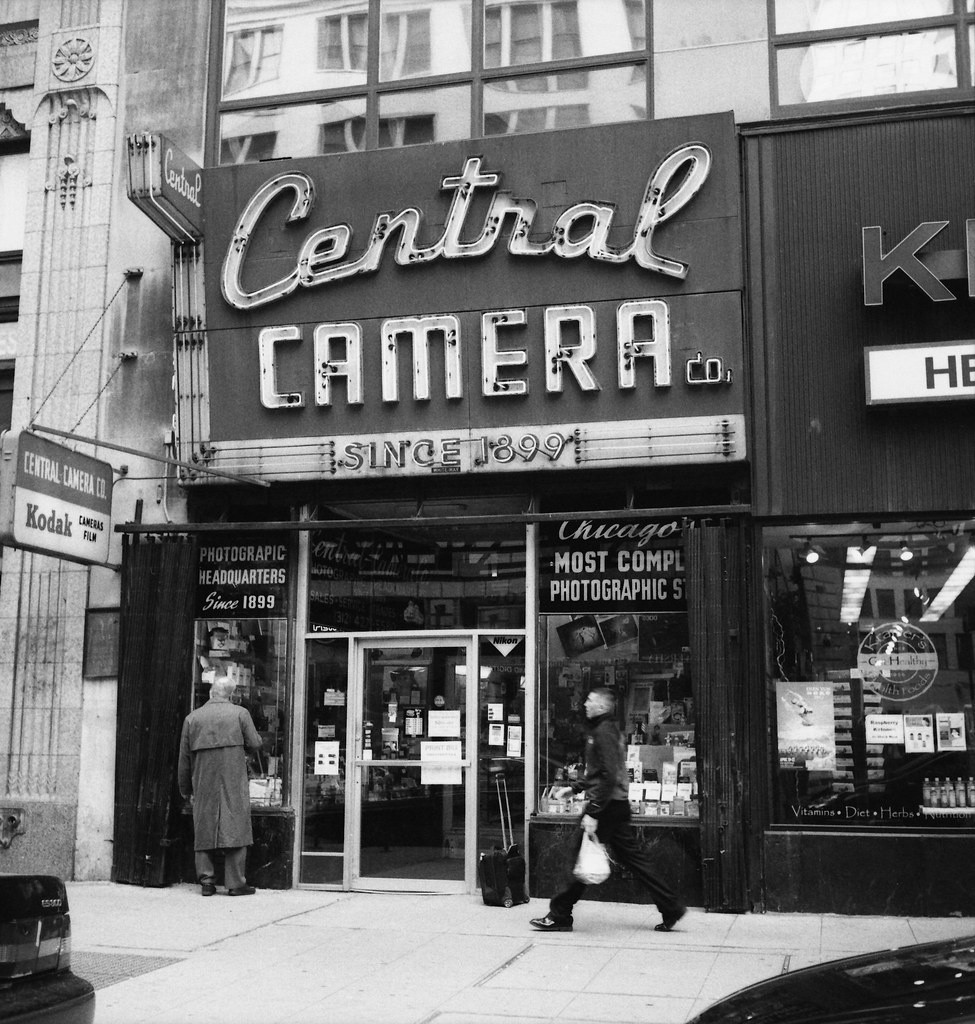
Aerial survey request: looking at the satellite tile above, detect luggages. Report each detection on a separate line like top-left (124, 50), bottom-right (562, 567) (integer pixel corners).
top-left (479, 773), bottom-right (530, 908)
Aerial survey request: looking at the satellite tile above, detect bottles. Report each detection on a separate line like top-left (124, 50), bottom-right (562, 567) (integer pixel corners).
top-left (970, 786), bottom-right (975, 807)
top-left (955, 777), bottom-right (962, 806)
top-left (949, 786), bottom-right (956, 808)
top-left (930, 787), bottom-right (937, 806)
top-left (923, 778), bottom-right (932, 806)
top-left (940, 787), bottom-right (948, 808)
top-left (967, 777), bottom-right (975, 804)
top-left (932, 778), bottom-right (943, 806)
top-left (944, 778), bottom-right (953, 805)
top-left (959, 784), bottom-right (966, 808)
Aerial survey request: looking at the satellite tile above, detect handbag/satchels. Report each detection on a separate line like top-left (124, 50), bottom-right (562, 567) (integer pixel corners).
top-left (573, 831), bottom-right (611, 884)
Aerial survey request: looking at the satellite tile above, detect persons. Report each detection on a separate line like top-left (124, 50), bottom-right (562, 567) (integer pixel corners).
top-left (206, 628), bottom-right (228, 650)
top-left (178, 678), bottom-right (263, 896)
top-left (530, 688), bottom-right (691, 934)
top-left (384, 668), bottom-right (423, 703)
top-left (780, 689), bottom-right (816, 726)
top-left (951, 729), bottom-right (963, 747)
top-left (482, 670), bottom-right (520, 738)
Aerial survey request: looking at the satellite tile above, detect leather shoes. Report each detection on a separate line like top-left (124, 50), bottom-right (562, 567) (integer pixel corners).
top-left (654, 906), bottom-right (686, 931)
top-left (530, 917), bottom-right (572, 931)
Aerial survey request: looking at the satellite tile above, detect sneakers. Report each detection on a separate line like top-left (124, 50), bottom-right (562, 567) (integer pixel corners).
top-left (229, 884), bottom-right (256, 896)
top-left (202, 885), bottom-right (216, 896)
